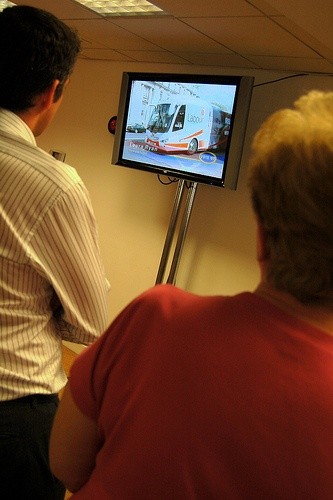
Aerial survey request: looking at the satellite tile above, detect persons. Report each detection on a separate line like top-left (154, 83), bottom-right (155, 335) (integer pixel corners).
top-left (49, 88), bottom-right (332, 500)
top-left (0, 3), bottom-right (113, 499)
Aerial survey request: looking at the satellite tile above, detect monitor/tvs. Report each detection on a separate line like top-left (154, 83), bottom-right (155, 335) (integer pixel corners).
top-left (109, 71), bottom-right (255, 195)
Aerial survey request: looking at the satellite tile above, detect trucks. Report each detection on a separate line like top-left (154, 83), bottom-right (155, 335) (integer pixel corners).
top-left (144, 96), bottom-right (232, 156)
